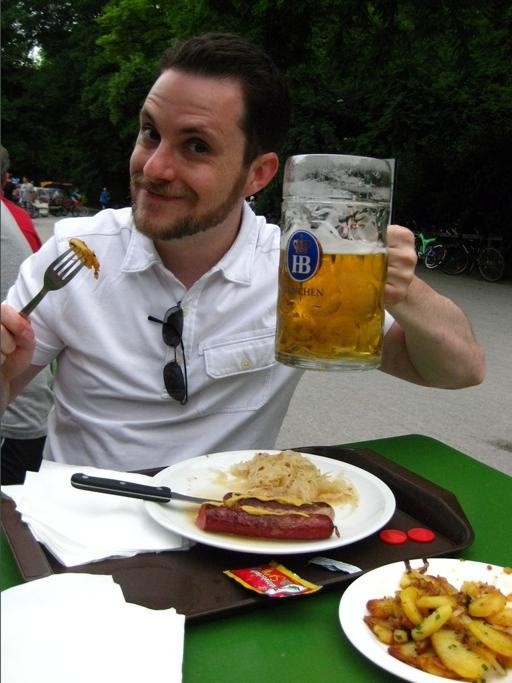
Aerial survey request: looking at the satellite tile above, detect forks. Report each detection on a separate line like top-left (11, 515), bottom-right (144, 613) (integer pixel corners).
top-left (19, 242), bottom-right (85, 321)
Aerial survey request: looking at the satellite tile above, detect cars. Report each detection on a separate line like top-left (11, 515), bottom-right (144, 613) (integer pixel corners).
top-left (15, 185), bottom-right (68, 218)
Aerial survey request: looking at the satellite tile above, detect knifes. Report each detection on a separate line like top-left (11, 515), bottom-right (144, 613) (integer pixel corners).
top-left (70, 471), bottom-right (223, 509)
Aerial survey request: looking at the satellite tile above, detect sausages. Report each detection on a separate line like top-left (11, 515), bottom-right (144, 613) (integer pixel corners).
top-left (196, 492), bottom-right (335, 539)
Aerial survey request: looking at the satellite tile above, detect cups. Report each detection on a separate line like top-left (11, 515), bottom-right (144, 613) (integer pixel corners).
top-left (270, 150), bottom-right (399, 374)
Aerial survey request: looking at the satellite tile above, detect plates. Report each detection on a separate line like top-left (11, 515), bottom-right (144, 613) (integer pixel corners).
top-left (144, 446), bottom-right (397, 560)
top-left (337, 552), bottom-right (511, 682)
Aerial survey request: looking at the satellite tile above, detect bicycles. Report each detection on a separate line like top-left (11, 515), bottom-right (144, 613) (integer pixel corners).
top-left (61, 197), bottom-right (90, 218)
top-left (408, 220), bottom-right (505, 282)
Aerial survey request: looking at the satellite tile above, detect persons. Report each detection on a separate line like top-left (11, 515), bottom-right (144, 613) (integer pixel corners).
top-left (0, 30), bottom-right (484, 483)
top-left (98, 185), bottom-right (111, 207)
top-left (1, 143), bottom-right (61, 483)
top-left (16, 174), bottom-right (38, 214)
top-left (4, 172), bottom-right (20, 199)
top-left (71, 185), bottom-right (82, 203)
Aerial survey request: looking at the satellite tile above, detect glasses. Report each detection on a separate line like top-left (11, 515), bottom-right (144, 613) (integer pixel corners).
top-left (148, 302), bottom-right (190, 406)
